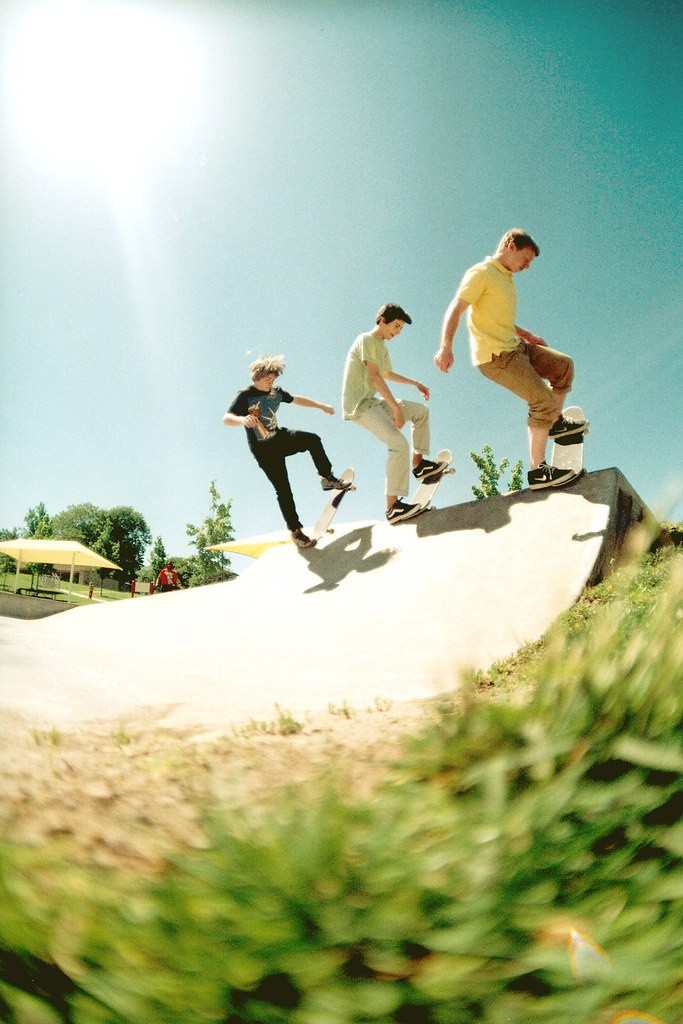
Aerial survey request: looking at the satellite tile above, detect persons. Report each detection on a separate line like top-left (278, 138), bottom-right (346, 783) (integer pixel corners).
top-left (222, 357), bottom-right (353, 549)
top-left (155, 561), bottom-right (180, 592)
top-left (341, 303), bottom-right (449, 525)
top-left (433, 229), bottom-right (589, 491)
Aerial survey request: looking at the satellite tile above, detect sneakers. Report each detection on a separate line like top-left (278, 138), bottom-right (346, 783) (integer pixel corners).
top-left (320, 476), bottom-right (351, 490)
top-left (548, 413), bottom-right (586, 439)
top-left (412, 459), bottom-right (449, 479)
top-left (385, 497), bottom-right (421, 524)
top-left (291, 530), bottom-right (317, 548)
top-left (528, 461), bottom-right (574, 490)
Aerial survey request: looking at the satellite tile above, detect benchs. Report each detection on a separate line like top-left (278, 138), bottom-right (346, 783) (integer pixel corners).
top-left (19, 587), bottom-right (64, 602)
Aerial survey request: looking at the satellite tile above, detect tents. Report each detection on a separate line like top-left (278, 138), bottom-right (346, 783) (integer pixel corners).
top-left (0, 538), bottom-right (124, 602)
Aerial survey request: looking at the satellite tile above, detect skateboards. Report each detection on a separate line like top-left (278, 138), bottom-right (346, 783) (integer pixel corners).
top-left (304, 467), bottom-right (357, 548)
top-left (400, 449), bottom-right (456, 520)
top-left (551, 406), bottom-right (589, 487)
